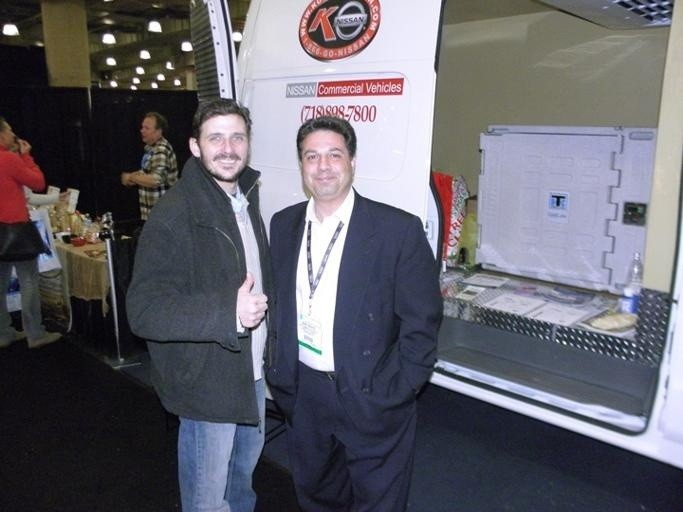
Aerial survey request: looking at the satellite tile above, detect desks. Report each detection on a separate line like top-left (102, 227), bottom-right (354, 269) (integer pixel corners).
top-left (40, 236), bottom-right (128, 317)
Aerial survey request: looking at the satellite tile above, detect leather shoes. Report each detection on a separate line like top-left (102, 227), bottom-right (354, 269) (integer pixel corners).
top-left (27, 332), bottom-right (61, 349)
top-left (0, 327), bottom-right (25, 347)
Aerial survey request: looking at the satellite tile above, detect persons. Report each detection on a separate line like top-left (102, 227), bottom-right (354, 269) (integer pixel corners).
top-left (119, 110), bottom-right (177, 224)
top-left (266, 115), bottom-right (442, 510)
top-left (0, 119), bottom-right (64, 349)
top-left (125, 97), bottom-right (280, 511)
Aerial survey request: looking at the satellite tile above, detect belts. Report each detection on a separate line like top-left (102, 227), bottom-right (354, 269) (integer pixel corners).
top-left (297, 360), bottom-right (338, 383)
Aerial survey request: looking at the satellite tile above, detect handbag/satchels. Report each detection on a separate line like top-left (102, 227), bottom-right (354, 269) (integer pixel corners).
top-left (11, 206), bottom-right (62, 278)
top-left (0, 220), bottom-right (52, 262)
top-left (4, 267), bottom-right (23, 313)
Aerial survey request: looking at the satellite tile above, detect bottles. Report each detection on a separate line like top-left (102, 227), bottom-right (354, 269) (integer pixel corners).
top-left (622, 251), bottom-right (643, 316)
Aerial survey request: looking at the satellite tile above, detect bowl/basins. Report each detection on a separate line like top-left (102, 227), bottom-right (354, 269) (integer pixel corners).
top-left (54, 230), bottom-right (87, 248)
